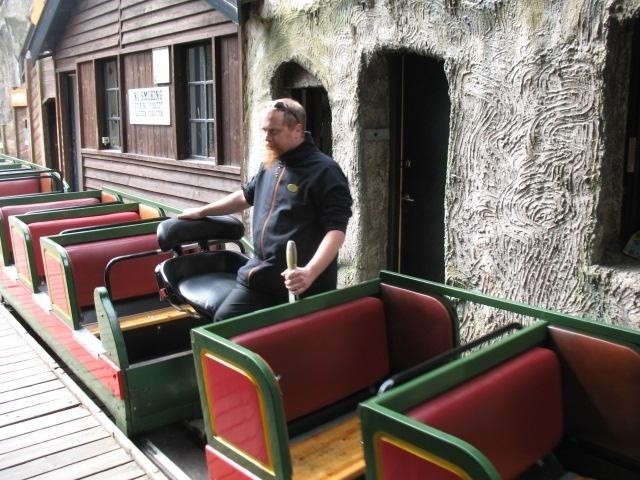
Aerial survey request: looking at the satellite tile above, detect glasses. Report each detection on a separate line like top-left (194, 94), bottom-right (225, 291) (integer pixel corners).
top-left (274, 101), bottom-right (300, 124)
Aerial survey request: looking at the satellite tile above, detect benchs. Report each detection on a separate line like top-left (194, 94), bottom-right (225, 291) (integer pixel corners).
top-left (190, 278), bottom-right (459, 479)
top-left (0, 163), bottom-right (199, 363)
top-left (357, 317), bottom-right (639, 477)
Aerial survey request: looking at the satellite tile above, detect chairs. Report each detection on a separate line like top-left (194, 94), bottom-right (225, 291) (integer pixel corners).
top-left (152, 213), bottom-right (251, 319)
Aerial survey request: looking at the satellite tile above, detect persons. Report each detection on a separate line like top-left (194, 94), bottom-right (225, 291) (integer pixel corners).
top-left (176, 98), bottom-right (352, 324)
